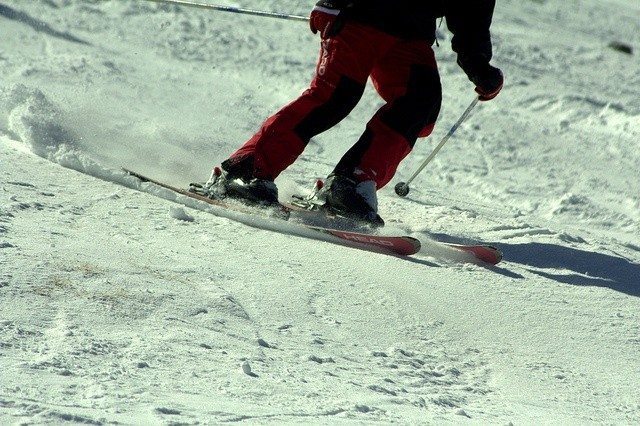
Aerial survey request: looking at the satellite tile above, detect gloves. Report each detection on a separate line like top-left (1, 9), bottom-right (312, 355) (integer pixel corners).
top-left (472, 66), bottom-right (504, 102)
top-left (309, 0), bottom-right (348, 39)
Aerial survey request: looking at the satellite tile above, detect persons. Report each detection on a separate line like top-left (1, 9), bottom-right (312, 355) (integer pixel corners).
top-left (220, 0), bottom-right (503, 226)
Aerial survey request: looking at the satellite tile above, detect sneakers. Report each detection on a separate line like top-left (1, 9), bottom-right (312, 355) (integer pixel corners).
top-left (216, 164), bottom-right (277, 203)
top-left (312, 175), bottom-right (376, 226)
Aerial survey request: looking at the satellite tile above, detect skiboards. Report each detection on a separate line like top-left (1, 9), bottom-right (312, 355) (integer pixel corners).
top-left (123, 167), bottom-right (503, 265)
